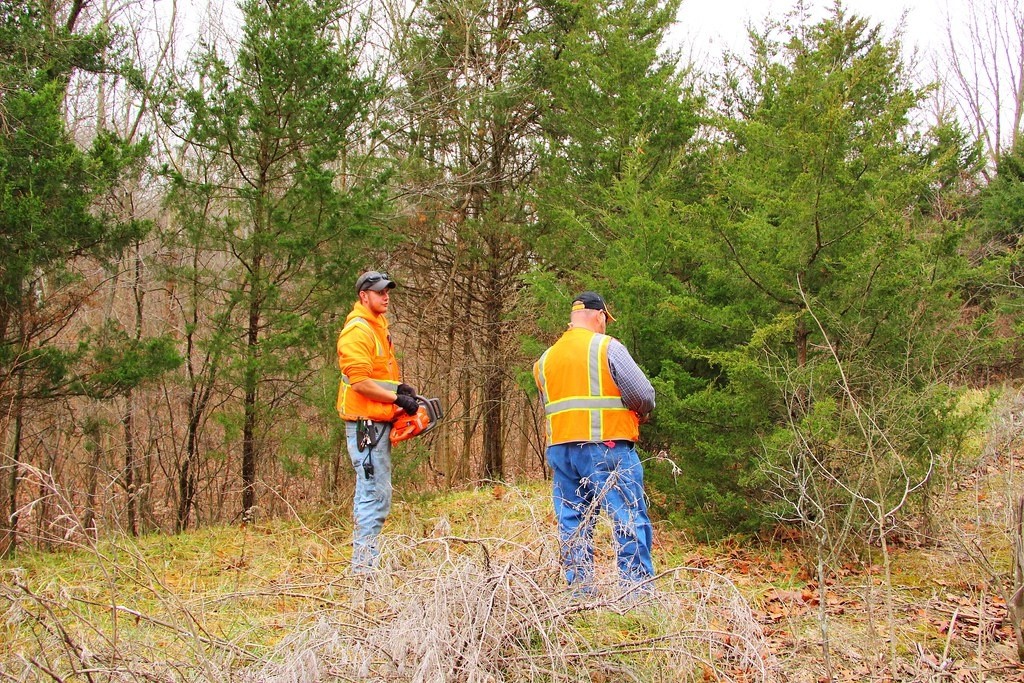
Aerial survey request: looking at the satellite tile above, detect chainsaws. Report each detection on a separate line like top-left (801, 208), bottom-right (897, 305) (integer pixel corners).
top-left (391, 394), bottom-right (444, 445)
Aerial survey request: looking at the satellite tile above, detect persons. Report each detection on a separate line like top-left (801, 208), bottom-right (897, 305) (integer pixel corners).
top-left (336, 271), bottom-right (419, 589)
top-left (530, 292), bottom-right (659, 604)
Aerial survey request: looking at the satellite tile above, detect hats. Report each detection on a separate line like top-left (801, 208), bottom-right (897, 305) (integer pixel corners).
top-left (355, 271), bottom-right (396, 292)
top-left (572, 291), bottom-right (616, 323)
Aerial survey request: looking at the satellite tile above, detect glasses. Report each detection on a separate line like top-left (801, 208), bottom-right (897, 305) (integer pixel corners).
top-left (358, 273), bottom-right (388, 294)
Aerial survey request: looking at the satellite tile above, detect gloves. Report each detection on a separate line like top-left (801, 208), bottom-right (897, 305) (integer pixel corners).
top-left (392, 383), bottom-right (420, 416)
